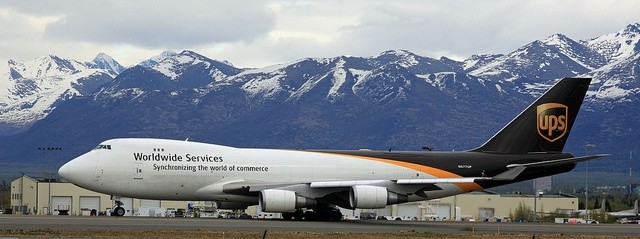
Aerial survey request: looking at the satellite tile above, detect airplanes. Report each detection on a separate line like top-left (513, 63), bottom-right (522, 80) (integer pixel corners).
top-left (58, 77), bottom-right (614, 220)
top-left (565, 199), bottom-right (640, 219)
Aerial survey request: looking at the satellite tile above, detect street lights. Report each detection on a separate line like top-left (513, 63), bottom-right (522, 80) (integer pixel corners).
top-left (584, 142), bottom-right (597, 222)
